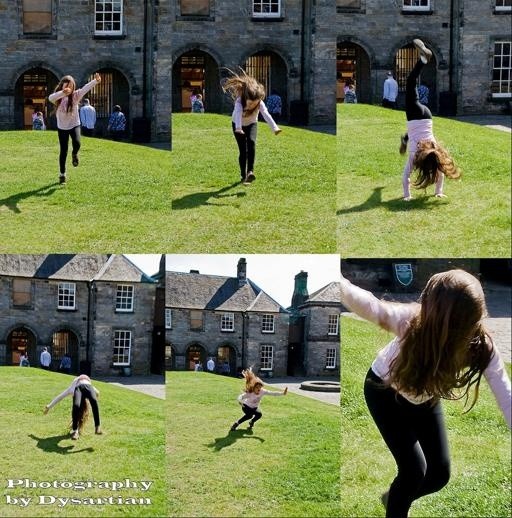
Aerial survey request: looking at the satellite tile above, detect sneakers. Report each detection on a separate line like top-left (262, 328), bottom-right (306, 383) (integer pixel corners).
top-left (410, 34), bottom-right (434, 64)
top-left (58, 172), bottom-right (67, 187)
top-left (71, 151), bottom-right (81, 168)
top-left (400, 137), bottom-right (407, 154)
top-left (244, 170), bottom-right (257, 187)
top-left (248, 420), bottom-right (255, 429)
top-left (70, 430), bottom-right (81, 441)
top-left (230, 422), bottom-right (239, 432)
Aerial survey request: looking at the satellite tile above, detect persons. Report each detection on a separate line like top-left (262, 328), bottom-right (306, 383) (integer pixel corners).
top-left (194, 360), bottom-right (200, 371)
top-left (79, 97), bottom-right (97, 137)
top-left (40, 346), bottom-right (52, 369)
top-left (220, 64), bottom-right (282, 184)
top-left (60, 352), bottom-right (72, 372)
top-left (20, 355), bottom-right (30, 367)
top-left (397, 37), bottom-right (462, 202)
top-left (206, 357), bottom-right (215, 373)
top-left (265, 87), bottom-right (282, 123)
top-left (19, 351), bottom-right (29, 365)
top-left (344, 85), bottom-right (355, 103)
top-left (33, 111), bottom-right (44, 130)
top-left (341, 268), bottom-right (512, 517)
top-left (107, 104), bottom-right (127, 138)
top-left (191, 93), bottom-right (203, 112)
top-left (47, 71), bottom-right (102, 180)
top-left (224, 361), bottom-right (231, 375)
top-left (197, 362), bottom-right (204, 371)
top-left (230, 368), bottom-right (289, 436)
top-left (380, 70), bottom-right (399, 108)
top-left (32, 107), bottom-right (47, 130)
top-left (342, 77), bottom-right (357, 103)
top-left (189, 87), bottom-right (206, 113)
top-left (416, 78), bottom-right (431, 108)
top-left (42, 371), bottom-right (105, 441)
